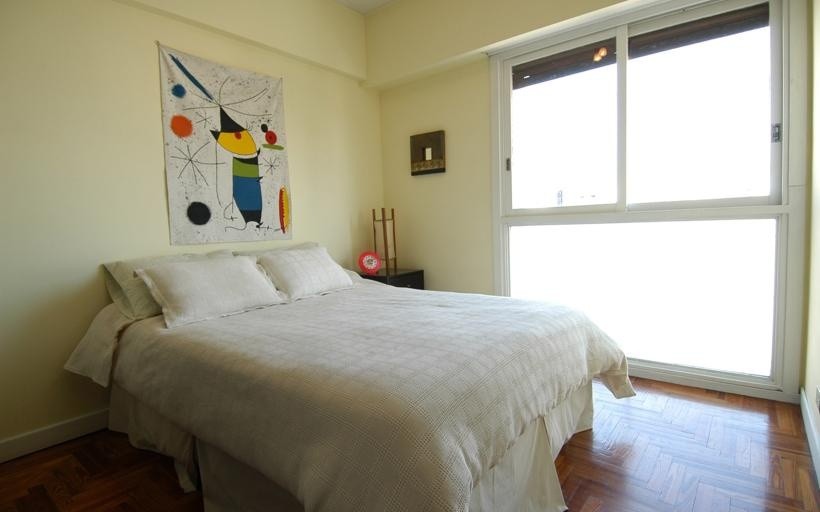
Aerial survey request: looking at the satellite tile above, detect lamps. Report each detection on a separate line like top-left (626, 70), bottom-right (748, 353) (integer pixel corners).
top-left (371, 206), bottom-right (398, 275)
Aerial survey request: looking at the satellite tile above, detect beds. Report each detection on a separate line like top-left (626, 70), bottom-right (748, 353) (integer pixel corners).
top-left (89, 243), bottom-right (593, 510)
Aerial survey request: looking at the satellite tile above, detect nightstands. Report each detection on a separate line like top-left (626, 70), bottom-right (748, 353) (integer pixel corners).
top-left (359, 267), bottom-right (425, 289)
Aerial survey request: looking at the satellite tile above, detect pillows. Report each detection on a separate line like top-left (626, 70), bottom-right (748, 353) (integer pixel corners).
top-left (100, 242), bottom-right (355, 329)
